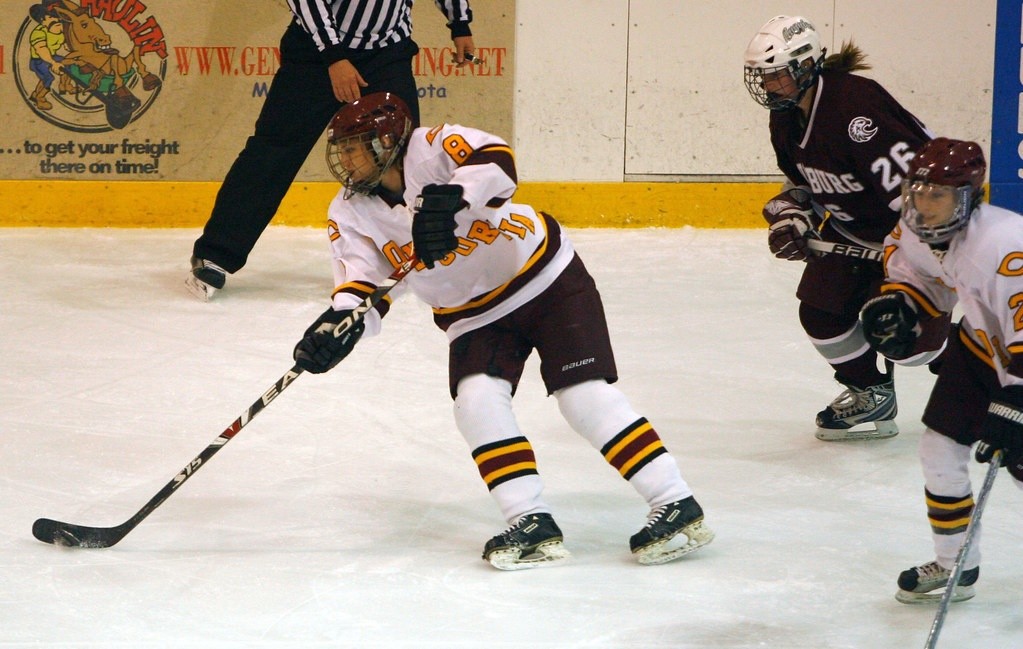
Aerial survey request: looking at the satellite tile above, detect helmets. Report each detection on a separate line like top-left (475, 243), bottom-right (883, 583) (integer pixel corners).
top-left (741, 14), bottom-right (829, 111)
top-left (909, 137), bottom-right (986, 245)
top-left (324, 93), bottom-right (412, 198)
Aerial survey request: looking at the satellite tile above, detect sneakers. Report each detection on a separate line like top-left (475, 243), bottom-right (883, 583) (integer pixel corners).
top-left (186, 252), bottom-right (225, 300)
top-left (894, 555), bottom-right (980, 606)
top-left (481, 513), bottom-right (572, 570)
top-left (816, 360), bottom-right (899, 441)
top-left (630, 496), bottom-right (715, 566)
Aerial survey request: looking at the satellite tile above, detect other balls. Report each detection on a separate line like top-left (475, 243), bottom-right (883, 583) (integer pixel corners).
top-left (56, 529), bottom-right (82, 547)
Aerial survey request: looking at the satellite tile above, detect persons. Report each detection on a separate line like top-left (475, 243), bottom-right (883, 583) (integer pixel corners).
top-left (185, 0), bottom-right (476, 300)
top-left (293, 91), bottom-right (714, 571)
top-left (743, 15), bottom-right (936, 441)
top-left (856, 136), bottom-right (1023, 604)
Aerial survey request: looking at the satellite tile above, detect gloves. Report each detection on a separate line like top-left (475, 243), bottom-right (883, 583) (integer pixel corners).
top-left (762, 191), bottom-right (825, 261)
top-left (975, 386), bottom-right (1023, 468)
top-left (293, 306), bottom-right (366, 374)
top-left (411, 183), bottom-right (464, 270)
top-left (859, 293), bottom-right (916, 360)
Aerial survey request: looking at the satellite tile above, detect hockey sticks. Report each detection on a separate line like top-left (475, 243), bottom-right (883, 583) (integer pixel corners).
top-left (32, 254), bottom-right (421, 550)
top-left (924, 448), bottom-right (1005, 649)
top-left (808, 238), bottom-right (883, 263)
